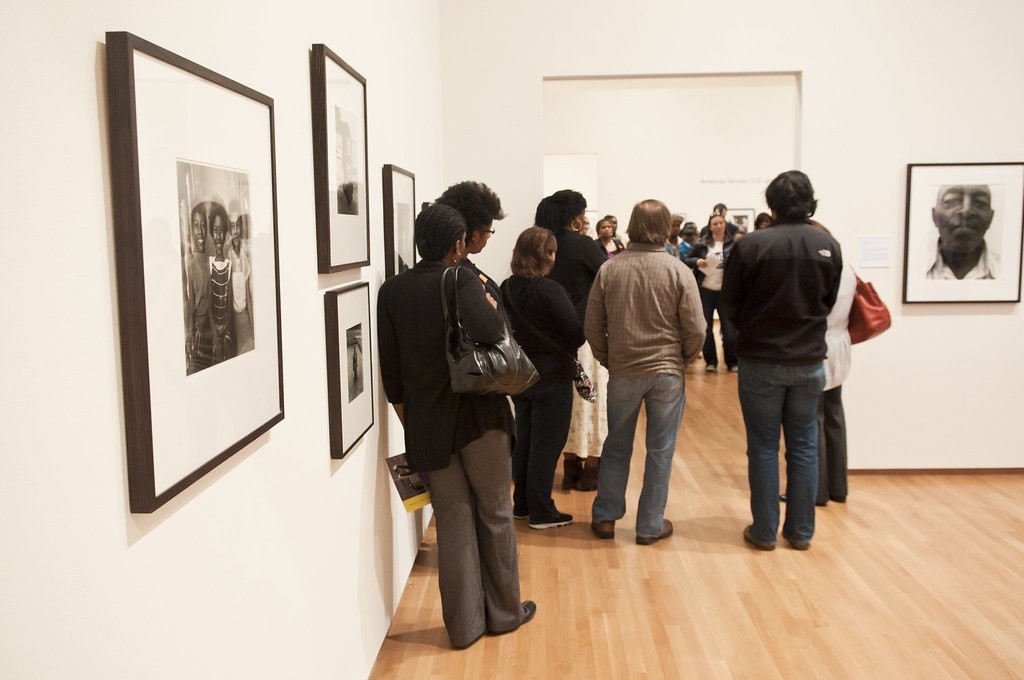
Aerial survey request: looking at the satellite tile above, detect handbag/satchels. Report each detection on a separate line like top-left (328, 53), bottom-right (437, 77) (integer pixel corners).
top-left (439, 265), bottom-right (542, 398)
top-left (574, 359), bottom-right (597, 404)
top-left (848, 260), bottom-right (892, 345)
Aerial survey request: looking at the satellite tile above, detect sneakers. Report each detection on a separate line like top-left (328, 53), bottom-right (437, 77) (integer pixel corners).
top-left (636, 518), bottom-right (674, 546)
top-left (513, 506), bottom-right (574, 530)
top-left (590, 519), bottom-right (616, 539)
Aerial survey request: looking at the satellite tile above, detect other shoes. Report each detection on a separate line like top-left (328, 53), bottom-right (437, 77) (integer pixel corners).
top-left (743, 525), bottom-right (775, 551)
top-left (520, 600), bottom-right (537, 624)
top-left (705, 364), bottom-right (716, 373)
top-left (781, 530), bottom-right (810, 551)
top-left (730, 364), bottom-right (739, 373)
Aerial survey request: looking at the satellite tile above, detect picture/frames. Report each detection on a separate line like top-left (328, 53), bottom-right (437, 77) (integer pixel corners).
top-left (902, 161), bottom-right (1024, 305)
top-left (381, 161), bottom-right (418, 281)
top-left (309, 39), bottom-right (372, 275)
top-left (324, 281), bottom-right (375, 462)
top-left (105, 30), bottom-right (286, 516)
top-left (724, 208), bottom-right (756, 235)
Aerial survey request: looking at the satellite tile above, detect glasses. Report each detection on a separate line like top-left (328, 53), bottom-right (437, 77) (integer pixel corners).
top-left (543, 229), bottom-right (555, 254)
top-left (709, 212), bottom-right (721, 217)
top-left (478, 227), bottom-right (496, 235)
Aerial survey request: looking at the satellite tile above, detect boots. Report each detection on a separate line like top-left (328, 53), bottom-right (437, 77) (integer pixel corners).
top-left (561, 458), bottom-right (596, 492)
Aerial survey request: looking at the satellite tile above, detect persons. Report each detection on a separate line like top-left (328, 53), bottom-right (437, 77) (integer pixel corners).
top-left (722, 170), bottom-right (843, 551)
top-left (594, 219), bottom-right (625, 257)
top-left (377, 204), bottom-right (537, 650)
top-left (499, 226), bottom-right (587, 529)
top-left (352, 345), bottom-right (358, 385)
top-left (683, 214), bottom-right (739, 372)
top-left (755, 213), bottom-right (771, 229)
top-left (924, 184), bottom-right (1001, 280)
top-left (701, 203), bottom-right (745, 242)
top-left (604, 215), bottom-right (624, 249)
top-left (667, 215), bottom-right (690, 258)
top-left (680, 222), bottom-right (700, 244)
top-left (187, 193), bottom-right (255, 375)
top-left (585, 197), bottom-right (707, 545)
top-left (779, 220), bottom-right (857, 506)
top-left (534, 189), bottom-right (608, 491)
top-left (433, 179), bottom-right (511, 333)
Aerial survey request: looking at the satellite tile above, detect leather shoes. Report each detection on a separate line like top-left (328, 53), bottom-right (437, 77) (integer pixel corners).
top-left (780, 494), bottom-right (827, 506)
top-left (829, 495), bottom-right (847, 503)
